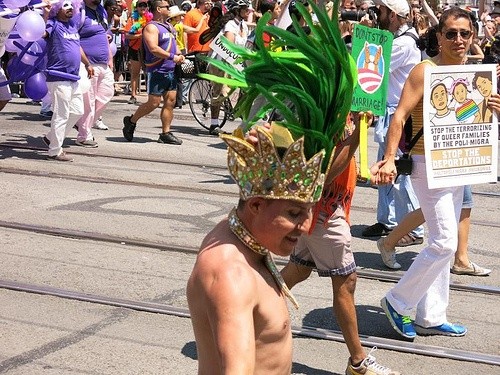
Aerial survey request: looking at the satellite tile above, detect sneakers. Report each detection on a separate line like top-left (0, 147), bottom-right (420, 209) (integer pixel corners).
top-left (94, 120), bottom-right (107, 130)
top-left (40, 110), bottom-right (54, 117)
top-left (346, 346), bottom-right (399, 375)
top-left (413, 320), bottom-right (467, 336)
top-left (158, 133), bottom-right (183, 144)
top-left (75, 138), bottom-right (98, 148)
top-left (43, 136), bottom-right (49, 147)
top-left (122, 114), bottom-right (136, 142)
top-left (47, 151), bottom-right (73, 162)
top-left (381, 296), bottom-right (416, 338)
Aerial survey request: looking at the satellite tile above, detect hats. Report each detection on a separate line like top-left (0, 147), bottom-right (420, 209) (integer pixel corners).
top-left (379, 0), bottom-right (410, 19)
top-left (197, 0), bottom-right (354, 203)
top-left (488, 8), bottom-right (500, 16)
top-left (136, 0), bottom-right (148, 7)
top-left (167, 6), bottom-right (186, 20)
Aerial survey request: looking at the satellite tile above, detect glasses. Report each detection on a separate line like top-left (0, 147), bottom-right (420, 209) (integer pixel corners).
top-left (158, 6), bottom-right (170, 11)
top-left (107, 8), bottom-right (117, 11)
top-left (441, 32), bottom-right (472, 41)
top-left (138, 5), bottom-right (147, 8)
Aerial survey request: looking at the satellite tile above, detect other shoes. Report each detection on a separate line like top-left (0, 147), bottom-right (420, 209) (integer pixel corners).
top-left (362, 223), bottom-right (395, 236)
top-left (394, 235), bottom-right (424, 246)
top-left (209, 124), bottom-right (232, 135)
top-left (377, 238), bottom-right (401, 269)
top-left (451, 262), bottom-right (491, 275)
top-left (128, 97), bottom-right (136, 104)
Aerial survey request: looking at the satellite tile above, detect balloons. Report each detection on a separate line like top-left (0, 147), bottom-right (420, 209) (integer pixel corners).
top-left (6, 38), bottom-right (49, 83)
top-left (24, 71), bottom-right (49, 101)
top-left (2, 0), bottom-right (31, 8)
top-left (109, 41), bottom-right (117, 56)
top-left (106, 30), bottom-right (114, 43)
top-left (5, 31), bottom-right (24, 52)
top-left (16, 11), bottom-right (46, 42)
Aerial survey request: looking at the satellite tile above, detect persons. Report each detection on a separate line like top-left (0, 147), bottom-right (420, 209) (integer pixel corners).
top-left (377, 24), bottom-right (500, 277)
top-left (185, 127), bottom-right (337, 375)
top-left (42, 0), bottom-right (249, 162)
top-left (0, 6), bottom-right (12, 111)
top-left (207, 0), bottom-right (500, 143)
top-left (373, 7), bottom-right (499, 341)
top-left (275, 25), bottom-right (400, 374)
top-left (360, 0), bottom-right (424, 246)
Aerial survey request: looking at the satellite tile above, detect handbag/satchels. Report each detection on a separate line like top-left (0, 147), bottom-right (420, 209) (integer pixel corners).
top-left (398, 157), bottom-right (412, 175)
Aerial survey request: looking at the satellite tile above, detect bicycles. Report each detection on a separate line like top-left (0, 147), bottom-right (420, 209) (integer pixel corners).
top-left (188, 71), bottom-right (244, 131)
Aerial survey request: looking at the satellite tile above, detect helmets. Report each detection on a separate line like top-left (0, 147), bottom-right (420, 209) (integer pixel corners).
top-left (228, 0), bottom-right (249, 12)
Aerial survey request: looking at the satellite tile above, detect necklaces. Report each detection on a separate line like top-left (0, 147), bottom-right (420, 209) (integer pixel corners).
top-left (227, 206), bottom-right (300, 310)
top-left (129, 10), bottom-right (153, 34)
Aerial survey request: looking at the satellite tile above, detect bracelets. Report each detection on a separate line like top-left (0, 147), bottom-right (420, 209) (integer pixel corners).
top-left (168, 53), bottom-right (174, 60)
